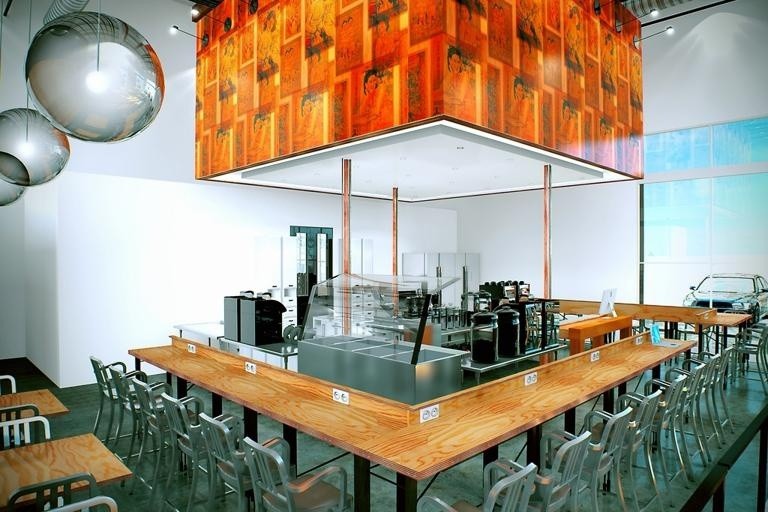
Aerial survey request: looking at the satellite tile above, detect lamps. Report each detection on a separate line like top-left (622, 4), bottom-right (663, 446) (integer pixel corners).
top-left (189, 5), bottom-right (234, 31)
top-left (22, 3), bottom-right (169, 147)
top-left (0, 2), bottom-right (72, 187)
top-left (0, 149), bottom-right (31, 209)
top-left (633, 25), bottom-right (678, 45)
top-left (611, 8), bottom-right (661, 35)
top-left (167, 23), bottom-right (215, 53)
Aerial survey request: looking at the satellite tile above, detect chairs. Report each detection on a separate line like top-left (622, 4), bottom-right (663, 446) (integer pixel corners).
top-left (416, 462), bottom-right (539, 512)
top-left (651, 309), bottom-right (716, 363)
top-left (198, 413), bottom-right (297, 512)
top-left (585, 346), bottom-right (734, 511)
top-left (6, 472), bottom-right (118, 512)
top-left (548, 407), bottom-right (634, 512)
top-left (88, 356), bottom-right (244, 511)
top-left (1, 374), bottom-right (16, 394)
top-left (487, 430), bottom-right (592, 511)
top-left (721, 310), bottom-right (768, 396)
top-left (0, 404), bottom-right (51, 450)
top-left (243, 436), bottom-right (354, 511)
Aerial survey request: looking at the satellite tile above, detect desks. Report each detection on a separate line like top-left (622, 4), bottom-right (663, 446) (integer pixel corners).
top-left (1, 432), bottom-right (133, 512)
top-left (1, 389), bottom-right (70, 425)
